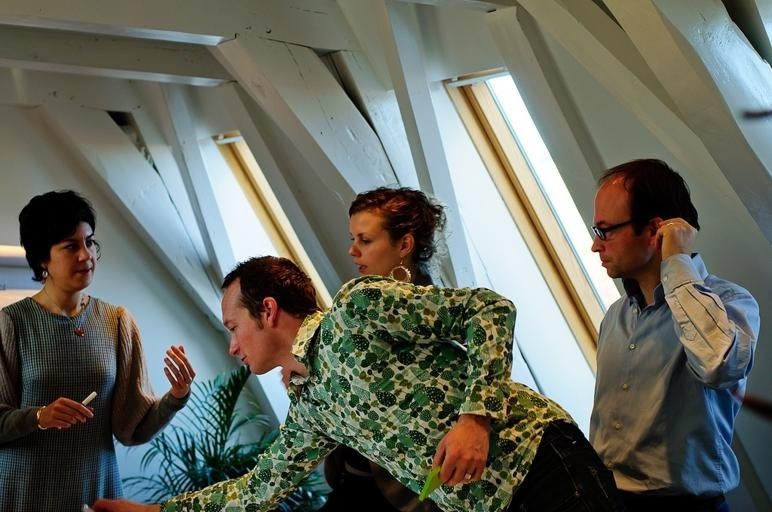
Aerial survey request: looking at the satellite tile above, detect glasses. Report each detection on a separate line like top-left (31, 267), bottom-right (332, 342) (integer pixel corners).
top-left (591, 219), bottom-right (632, 241)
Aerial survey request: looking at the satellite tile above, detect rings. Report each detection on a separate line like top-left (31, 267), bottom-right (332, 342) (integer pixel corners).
top-left (464, 473), bottom-right (471, 479)
top-left (666, 223), bottom-right (672, 225)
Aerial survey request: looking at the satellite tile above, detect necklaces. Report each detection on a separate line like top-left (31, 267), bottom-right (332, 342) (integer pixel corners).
top-left (43, 285), bottom-right (87, 338)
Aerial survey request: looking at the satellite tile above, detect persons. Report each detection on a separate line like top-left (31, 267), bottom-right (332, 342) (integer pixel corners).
top-left (589, 156), bottom-right (761, 512)
top-left (0, 188), bottom-right (200, 512)
top-left (311, 187), bottom-right (449, 511)
top-left (90, 255), bottom-right (621, 511)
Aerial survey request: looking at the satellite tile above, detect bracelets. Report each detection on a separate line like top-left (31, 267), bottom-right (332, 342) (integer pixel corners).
top-left (36, 405), bottom-right (48, 431)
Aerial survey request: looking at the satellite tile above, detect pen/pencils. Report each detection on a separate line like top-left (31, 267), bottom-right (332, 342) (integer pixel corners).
top-left (58, 391), bottom-right (98, 430)
